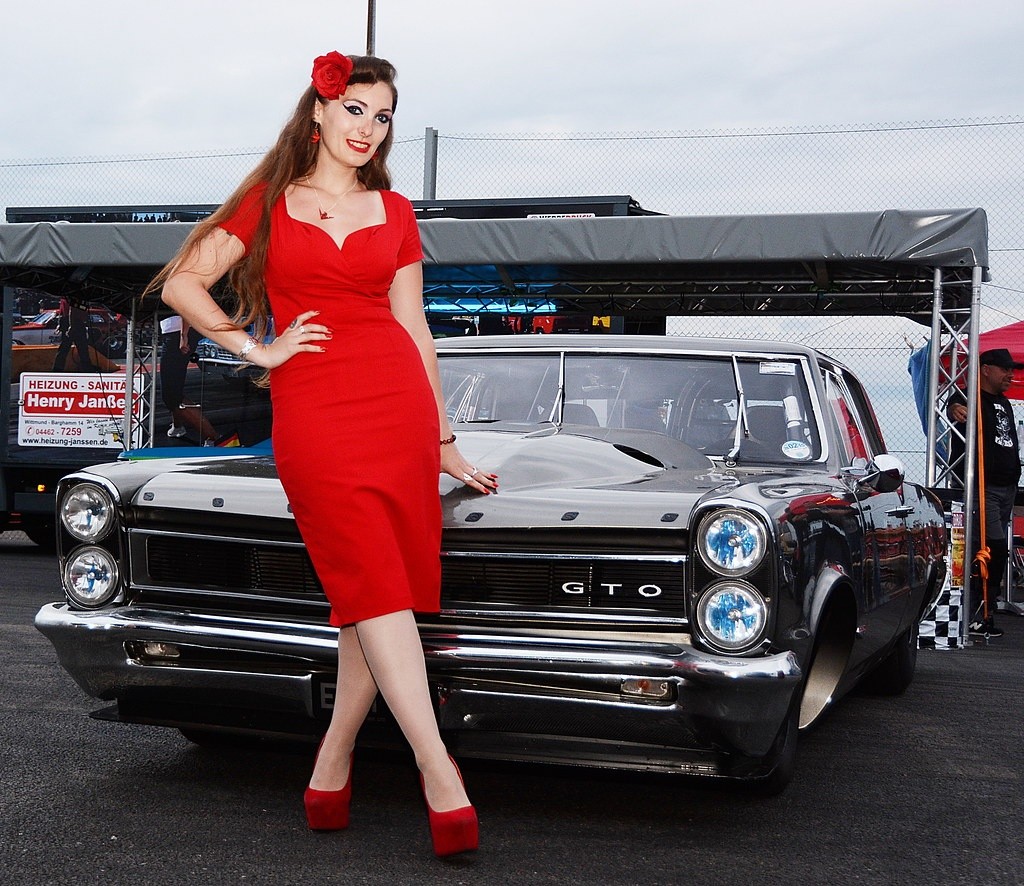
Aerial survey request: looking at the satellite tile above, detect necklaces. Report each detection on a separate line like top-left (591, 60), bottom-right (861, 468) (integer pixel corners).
top-left (304, 171), bottom-right (359, 221)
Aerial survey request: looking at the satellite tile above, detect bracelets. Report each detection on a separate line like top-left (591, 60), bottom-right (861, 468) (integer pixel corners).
top-left (439, 433), bottom-right (457, 446)
top-left (237, 336), bottom-right (259, 362)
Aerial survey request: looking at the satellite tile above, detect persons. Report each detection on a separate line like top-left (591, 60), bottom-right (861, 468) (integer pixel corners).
top-left (48, 295), bottom-right (97, 374)
top-left (140, 50), bottom-right (501, 859)
top-left (154, 294), bottom-right (228, 449)
top-left (15, 292), bottom-right (30, 326)
top-left (945, 347), bottom-right (1022, 640)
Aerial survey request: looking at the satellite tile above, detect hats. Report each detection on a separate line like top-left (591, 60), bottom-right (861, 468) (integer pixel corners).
top-left (980, 349), bottom-right (1024, 370)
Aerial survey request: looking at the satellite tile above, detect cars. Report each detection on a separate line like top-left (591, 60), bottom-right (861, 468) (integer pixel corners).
top-left (9, 307), bottom-right (271, 552)
top-left (30, 335), bottom-right (949, 814)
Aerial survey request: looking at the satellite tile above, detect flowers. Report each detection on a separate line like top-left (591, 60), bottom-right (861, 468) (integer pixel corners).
top-left (311, 50), bottom-right (356, 102)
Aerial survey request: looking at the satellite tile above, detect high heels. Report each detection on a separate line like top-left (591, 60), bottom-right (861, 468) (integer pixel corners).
top-left (303, 732), bottom-right (353, 828)
top-left (421, 754), bottom-right (478, 855)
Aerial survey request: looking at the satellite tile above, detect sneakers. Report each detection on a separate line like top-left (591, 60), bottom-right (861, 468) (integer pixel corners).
top-left (167, 422), bottom-right (187, 437)
top-left (203, 435), bottom-right (223, 448)
top-left (961, 620), bottom-right (1004, 636)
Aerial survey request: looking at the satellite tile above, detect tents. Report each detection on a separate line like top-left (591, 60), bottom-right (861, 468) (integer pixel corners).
top-left (937, 318), bottom-right (1023, 402)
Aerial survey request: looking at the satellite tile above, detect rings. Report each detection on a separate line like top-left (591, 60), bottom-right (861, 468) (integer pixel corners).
top-left (470, 466), bottom-right (480, 477)
top-left (462, 472), bottom-right (473, 483)
top-left (300, 325), bottom-right (305, 335)
